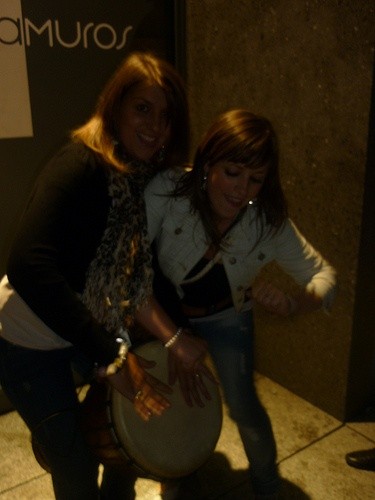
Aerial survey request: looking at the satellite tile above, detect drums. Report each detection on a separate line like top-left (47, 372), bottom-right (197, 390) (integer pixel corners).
top-left (102, 334), bottom-right (224, 484)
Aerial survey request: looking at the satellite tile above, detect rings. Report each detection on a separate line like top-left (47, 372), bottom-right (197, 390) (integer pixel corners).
top-left (135, 390), bottom-right (143, 398)
top-left (195, 374), bottom-right (200, 377)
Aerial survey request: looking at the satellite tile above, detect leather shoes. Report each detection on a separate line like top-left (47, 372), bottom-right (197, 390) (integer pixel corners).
top-left (345, 448), bottom-right (375, 471)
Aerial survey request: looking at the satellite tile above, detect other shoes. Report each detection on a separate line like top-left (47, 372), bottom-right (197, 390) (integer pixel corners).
top-left (162, 480), bottom-right (183, 500)
top-left (251, 492), bottom-right (279, 500)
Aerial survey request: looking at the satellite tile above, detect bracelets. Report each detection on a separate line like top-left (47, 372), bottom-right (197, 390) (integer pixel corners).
top-left (285, 294), bottom-right (295, 318)
top-left (96, 341), bottom-right (128, 379)
top-left (164, 328), bottom-right (181, 348)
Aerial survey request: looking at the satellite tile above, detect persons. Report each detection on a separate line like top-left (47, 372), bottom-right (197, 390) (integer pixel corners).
top-left (132, 109), bottom-right (337, 500)
top-left (1, 55), bottom-right (190, 500)
